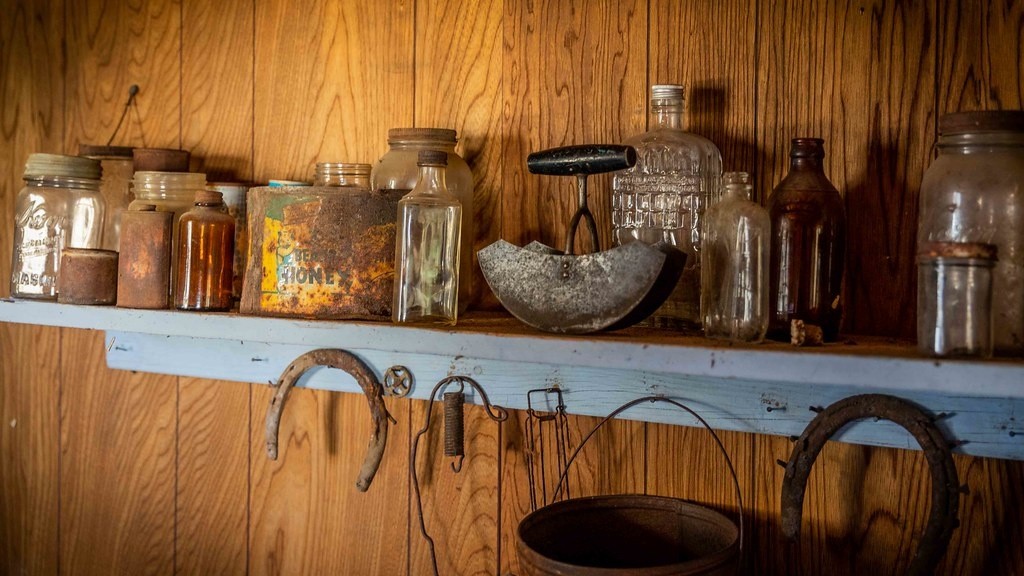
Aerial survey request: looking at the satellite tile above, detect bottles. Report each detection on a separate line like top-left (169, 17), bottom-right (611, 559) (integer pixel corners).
top-left (368, 126), bottom-right (476, 316)
top-left (914, 241), bottom-right (999, 359)
top-left (766, 136), bottom-right (843, 342)
top-left (310, 161), bottom-right (374, 188)
top-left (391, 148), bottom-right (459, 328)
top-left (7, 153), bottom-right (106, 303)
top-left (77, 142), bottom-right (140, 254)
top-left (611, 82), bottom-right (724, 332)
top-left (915, 105), bottom-right (1024, 355)
top-left (126, 170), bottom-right (211, 312)
top-left (696, 170), bottom-right (769, 341)
top-left (173, 188), bottom-right (234, 311)
